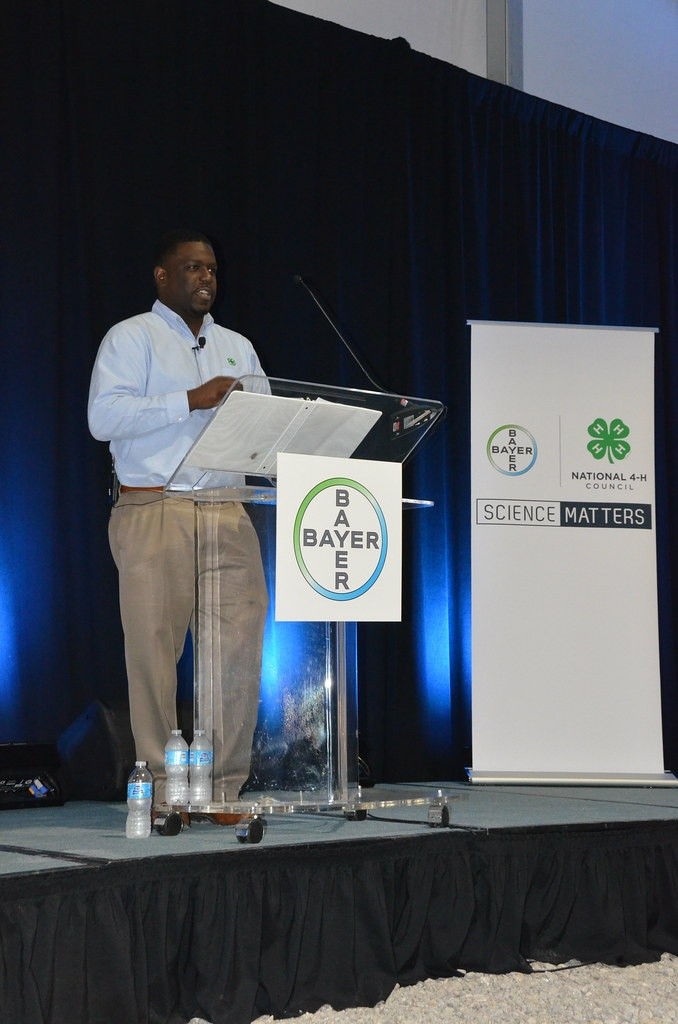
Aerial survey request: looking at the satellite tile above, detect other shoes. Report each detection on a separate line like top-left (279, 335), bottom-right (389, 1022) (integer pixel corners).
top-left (205, 812), bottom-right (260, 827)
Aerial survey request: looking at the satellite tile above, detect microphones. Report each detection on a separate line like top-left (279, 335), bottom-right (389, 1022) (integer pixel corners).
top-left (294, 276), bottom-right (436, 441)
top-left (193, 337), bottom-right (205, 350)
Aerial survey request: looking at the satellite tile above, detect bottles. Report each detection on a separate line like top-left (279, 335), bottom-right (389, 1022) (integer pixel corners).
top-left (189, 728), bottom-right (213, 805)
top-left (164, 729), bottom-right (189, 805)
top-left (126, 760), bottom-right (152, 838)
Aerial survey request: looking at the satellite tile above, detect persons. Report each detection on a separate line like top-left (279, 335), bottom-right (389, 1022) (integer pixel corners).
top-left (88, 232), bottom-right (292, 823)
